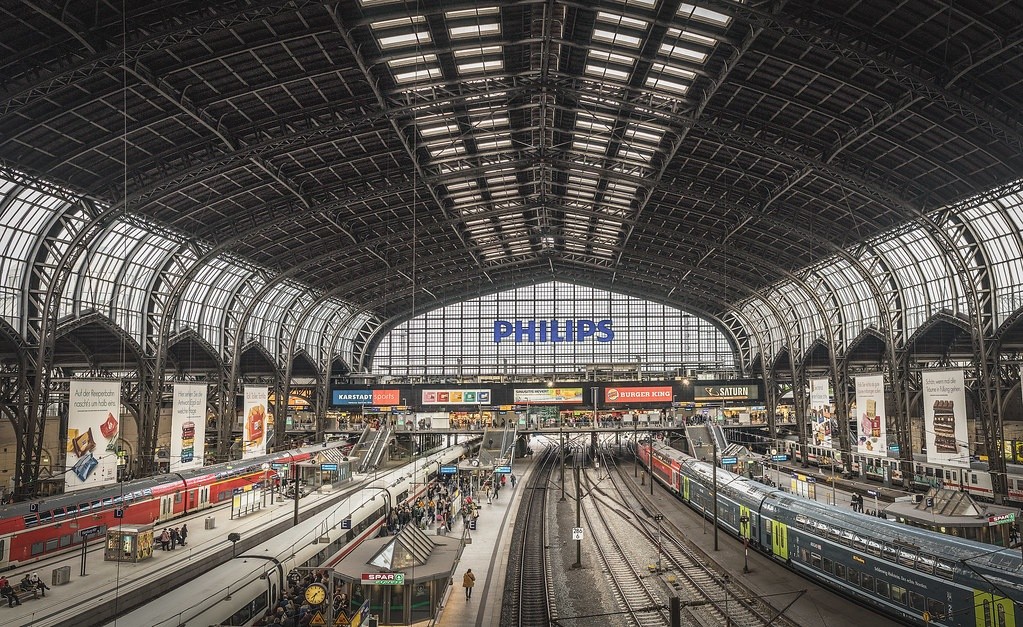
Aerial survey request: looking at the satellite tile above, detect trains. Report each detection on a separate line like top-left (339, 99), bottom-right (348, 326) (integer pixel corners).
top-left (0, 431), bottom-right (364, 575)
top-left (97, 433), bottom-right (485, 627)
top-left (635, 435), bottom-right (1023, 627)
top-left (723, 429), bottom-right (1023, 508)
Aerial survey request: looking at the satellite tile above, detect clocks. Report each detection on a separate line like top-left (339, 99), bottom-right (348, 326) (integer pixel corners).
top-left (471, 460), bottom-right (479, 467)
top-left (304, 582), bottom-right (328, 605)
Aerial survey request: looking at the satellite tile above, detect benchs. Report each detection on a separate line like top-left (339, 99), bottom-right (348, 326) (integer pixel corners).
top-left (0, 582), bottom-right (38, 606)
top-left (153, 535), bottom-right (162, 550)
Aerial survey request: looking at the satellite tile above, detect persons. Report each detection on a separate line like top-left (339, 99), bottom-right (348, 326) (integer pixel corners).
top-left (275, 475), bottom-right (280, 491)
top-left (850, 491), bottom-right (887, 519)
top-left (764, 474), bottom-right (777, 487)
top-left (510, 474), bottom-right (516, 487)
top-left (21, 573), bottom-right (51, 600)
top-left (778, 483), bottom-right (784, 490)
top-left (209, 416), bottom-right (216, 428)
top-left (489, 438), bottom-right (493, 447)
top-left (292, 413), bottom-right (431, 430)
top-left (282, 476), bottom-right (288, 488)
top-left (749, 470), bottom-right (754, 479)
top-left (450, 412), bottom-right (624, 429)
top-left (463, 569), bottom-right (476, 601)
top-left (1009, 522), bottom-right (1018, 544)
top-left (258, 570), bottom-right (330, 627)
top-left (696, 436), bottom-right (704, 445)
top-left (527, 446), bottom-right (530, 452)
top-left (159, 524), bottom-right (189, 551)
top-left (379, 469), bottom-right (506, 536)
top-left (662, 412), bottom-right (792, 426)
top-left (0, 575), bottom-right (22, 608)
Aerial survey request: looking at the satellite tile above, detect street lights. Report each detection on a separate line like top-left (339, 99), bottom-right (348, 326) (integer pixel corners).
top-left (403, 398), bottom-right (406, 430)
top-left (525, 397), bottom-right (529, 429)
top-left (673, 395), bottom-right (677, 427)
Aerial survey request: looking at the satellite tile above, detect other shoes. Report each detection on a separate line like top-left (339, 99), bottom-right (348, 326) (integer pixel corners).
top-left (9, 606), bottom-right (13, 608)
top-left (16, 603), bottom-right (22, 605)
top-left (42, 594), bottom-right (45, 596)
top-left (47, 588), bottom-right (50, 590)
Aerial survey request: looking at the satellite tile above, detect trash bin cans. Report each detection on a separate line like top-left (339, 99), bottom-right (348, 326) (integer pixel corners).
top-left (437, 525), bottom-right (446, 536)
top-left (352, 424), bottom-right (361, 431)
top-left (469, 425), bottom-right (475, 430)
top-left (204, 516), bottom-right (215, 530)
top-left (51, 566), bottom-right (71, 585)
